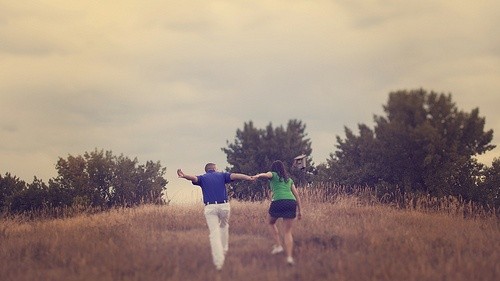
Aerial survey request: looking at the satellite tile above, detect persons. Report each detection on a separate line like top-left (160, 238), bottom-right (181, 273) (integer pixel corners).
top-left (177, 163), bottom-right (257, 272)
top-left (252, 160), bottom-right (303, 266)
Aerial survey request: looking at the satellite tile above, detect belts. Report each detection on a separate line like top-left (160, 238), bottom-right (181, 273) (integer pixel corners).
top-left (205, 200), bottom-right (228, 206)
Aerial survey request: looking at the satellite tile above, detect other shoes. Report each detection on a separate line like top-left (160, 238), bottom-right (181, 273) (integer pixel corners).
top-left (215, 265), bottom-right (223, 270)
top-left (286, 256), bottom-right (294, 264)
top-left (223, 249), bottom-right (228, 255)
top-left (271, 245), bottom-right (284, 255)
